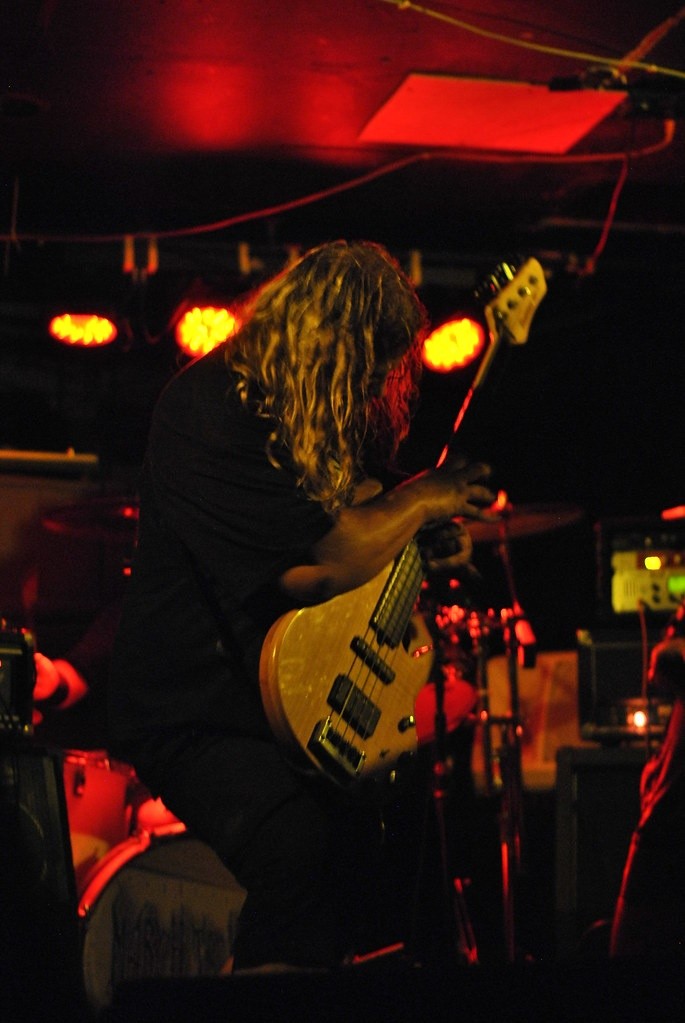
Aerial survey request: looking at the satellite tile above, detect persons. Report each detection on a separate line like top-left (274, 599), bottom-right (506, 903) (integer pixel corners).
top-left (107, 239), bottom-right (494, 970)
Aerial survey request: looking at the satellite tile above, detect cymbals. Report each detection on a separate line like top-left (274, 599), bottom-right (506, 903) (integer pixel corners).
top-left (449, 499), bottom-right (585, 542)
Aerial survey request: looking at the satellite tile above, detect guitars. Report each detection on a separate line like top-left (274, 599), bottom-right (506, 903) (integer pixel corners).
top-left (255, 253), bottom-right (551, 800)
